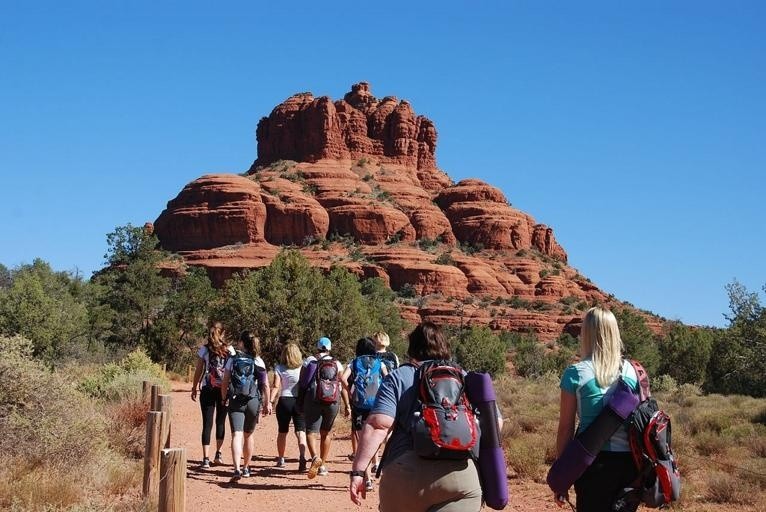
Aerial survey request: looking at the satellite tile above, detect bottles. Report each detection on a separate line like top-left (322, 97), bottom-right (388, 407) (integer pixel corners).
top-left (249, 379), bottom-right (258, 397)
top-left (202, 370), bottom-right (210, 385)
top-left (310, 378), bottom-right (317, 389)
top-left (411, 411), bottom-right (421, 427)
top-left (241, 375), bottom-right (246, 386)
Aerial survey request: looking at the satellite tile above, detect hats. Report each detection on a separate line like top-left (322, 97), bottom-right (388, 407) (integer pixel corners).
top-left (317, 337), bottom-right (332, 351)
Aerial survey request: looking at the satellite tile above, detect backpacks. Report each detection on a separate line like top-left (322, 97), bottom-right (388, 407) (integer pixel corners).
top-left (230, 353), bottom-right (258, 398)
top-left (386, 359), bottom-right (484, 459)
top-left (625, 359), bottom-right (681, 508)
top-left (204, 344), bottom-right (232, 390)
top-left (353, 354), bottom-right (383, 410)
top-left (313, 353), bottom-right (340, 403)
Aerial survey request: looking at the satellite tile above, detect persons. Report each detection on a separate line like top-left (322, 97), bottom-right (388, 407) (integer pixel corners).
top-left (191, 322), bottom-right (400, 492)
top-left (553, 307), bottom-right (651, 512)
top-left (350, 323), bottom-right (503, 512)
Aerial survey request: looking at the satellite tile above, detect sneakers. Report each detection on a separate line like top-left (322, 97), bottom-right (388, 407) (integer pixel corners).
top-left (364, 479), bottom-right (373, 490)
top-left (213, 454), bottom-right (223, 463)
top-left (299, 458), bottom-right (306, 470)
top-left (229, 470), bottom-right (241, 484)
top-left (348, 454), bottom-right (356, 461)
top-left (201, 459), bottom-right (210, 468)
top-left (242, 468), bottom-right (251, 477)
top-left (371, 465), bottom-right (379, 473)
top-left (317, 463), bottom-right (328, 476)
top-left (277, 457), bottom-right (284, 467)
top-left (308, 456), bottom-right (322, 479)
